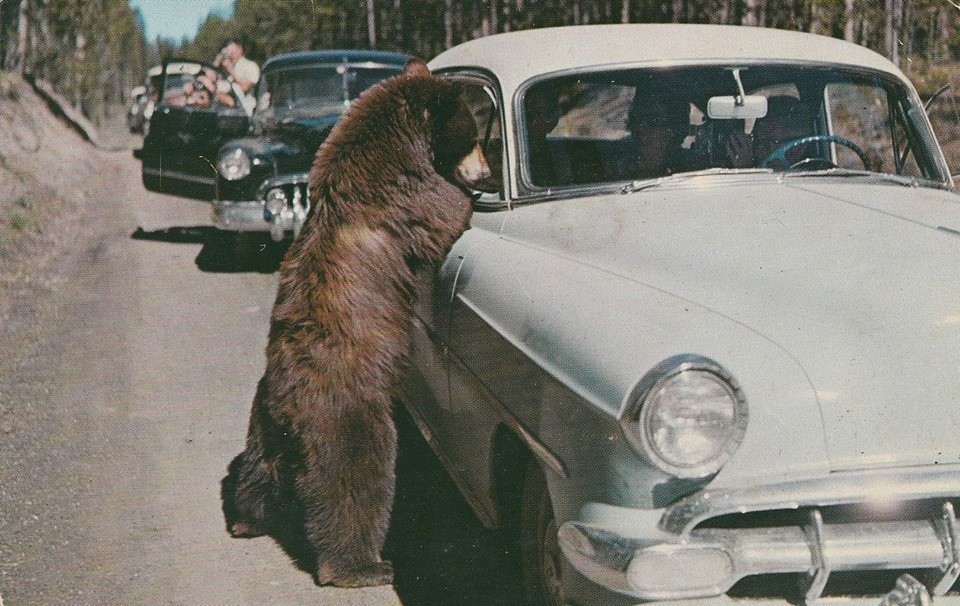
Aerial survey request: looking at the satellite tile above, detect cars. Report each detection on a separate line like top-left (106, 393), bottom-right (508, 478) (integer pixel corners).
top-left (129, 49), bottom-right (410, 275)
top-left (389, 22), bottom-right (960, 606)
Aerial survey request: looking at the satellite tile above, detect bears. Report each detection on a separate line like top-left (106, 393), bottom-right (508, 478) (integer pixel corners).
top-left (230, 56), bottom-right (491, 581)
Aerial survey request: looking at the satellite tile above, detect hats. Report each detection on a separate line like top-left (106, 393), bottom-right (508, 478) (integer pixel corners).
top-left (755, 93), bottom-right (802, 122)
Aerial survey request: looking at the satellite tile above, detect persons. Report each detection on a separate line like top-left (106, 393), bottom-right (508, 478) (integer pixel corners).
top-left (185, 35), bottom-right (273, 114)
top-left (488, 75), bottom-right (819, 196)
